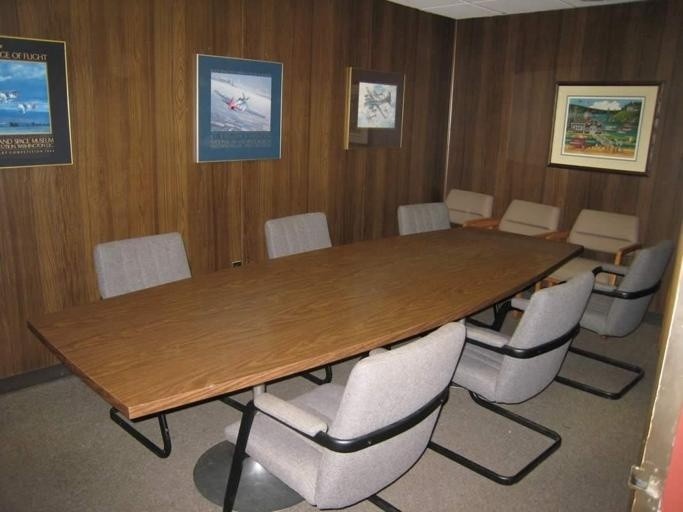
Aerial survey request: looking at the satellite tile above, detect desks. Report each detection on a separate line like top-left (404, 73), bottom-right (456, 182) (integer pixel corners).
top-left (26, 227), bottom-right (585, 511)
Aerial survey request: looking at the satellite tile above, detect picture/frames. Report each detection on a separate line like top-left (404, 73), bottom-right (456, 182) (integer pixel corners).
top-left (345, 66), bottom-right (407, 151)
top-left (196, 53), bottom-right (283, 164)
top-left (546, 80), bottom-right (665, 177)
top-left (0, 36), bottom-right (73, 169)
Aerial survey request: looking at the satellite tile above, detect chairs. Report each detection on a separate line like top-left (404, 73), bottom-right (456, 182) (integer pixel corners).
top-left (92, 232), bottom-right (245, 459)
top-left (443, 189), bottom-right (494, 231)
top-left (554, 240), bottom-right (674, 400)
top-left (411, 272), bottom-right (595, 486)
top-left (264, 212), bottom-right (391, 385)
top-left (535, 209), bottom-right (643, 294)
top-left (223, 321), bottom-right (466, 512)
top-left (397, 202), bottom-right (451, 236)
top-left (463, 199), bottom-right (561, 318)
